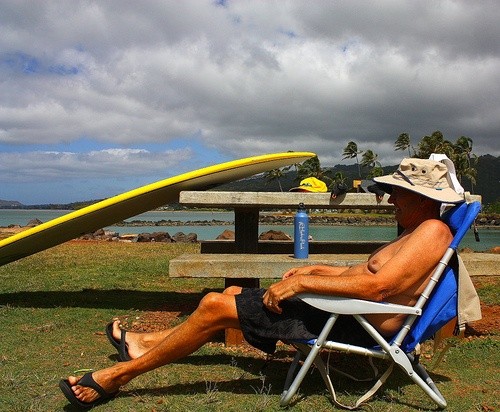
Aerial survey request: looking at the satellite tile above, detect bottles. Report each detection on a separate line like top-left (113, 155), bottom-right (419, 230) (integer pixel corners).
top-left (294, 203), bottom-right (308, 260)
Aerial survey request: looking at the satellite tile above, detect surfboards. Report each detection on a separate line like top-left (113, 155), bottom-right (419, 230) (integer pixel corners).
top-left (0, 151), bottom-right (317, 268)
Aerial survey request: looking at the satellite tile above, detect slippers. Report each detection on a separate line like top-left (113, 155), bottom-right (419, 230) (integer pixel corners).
top-left (106, 321), bottom-right (132, 362)
top-left (59, 373), bottom-right (113, 411)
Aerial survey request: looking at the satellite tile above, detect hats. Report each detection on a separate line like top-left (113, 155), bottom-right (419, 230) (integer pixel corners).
top-left (289, 177), bottom-right (327, 193)
top-left (373, 157), bottom-right (464, 203)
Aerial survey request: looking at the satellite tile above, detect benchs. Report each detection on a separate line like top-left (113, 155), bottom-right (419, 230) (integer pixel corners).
top-left (169, 253), bottom-right (481, 347)
top-left (199, 239), bottom-right (390, 253)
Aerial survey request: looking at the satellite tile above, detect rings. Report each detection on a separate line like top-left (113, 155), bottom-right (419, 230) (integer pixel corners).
top-left (268, 301), bottom-right (273, 303)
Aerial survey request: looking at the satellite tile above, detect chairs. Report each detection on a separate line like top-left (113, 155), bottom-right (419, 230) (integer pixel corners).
top-left (279, 197), bottom-right (482, 406)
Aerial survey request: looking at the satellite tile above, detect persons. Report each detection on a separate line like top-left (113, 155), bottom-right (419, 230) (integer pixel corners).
top-left (58, 158), bottom-right (465, 409)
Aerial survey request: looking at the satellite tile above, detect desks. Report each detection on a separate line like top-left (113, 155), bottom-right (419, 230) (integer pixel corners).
top-left (178, 190), bottom-right (482, 290)
